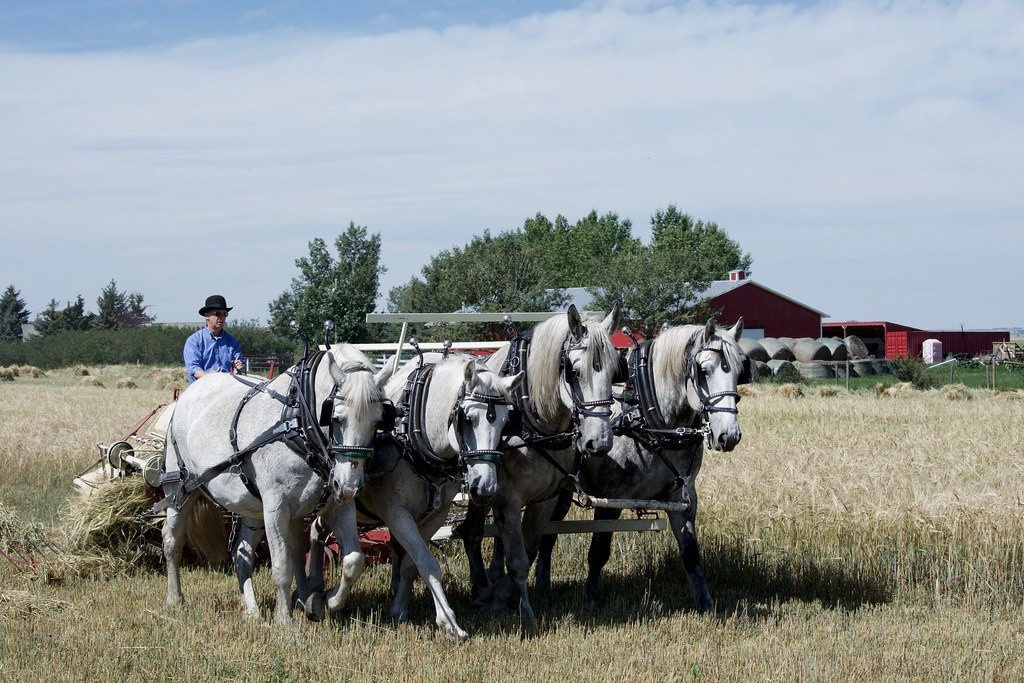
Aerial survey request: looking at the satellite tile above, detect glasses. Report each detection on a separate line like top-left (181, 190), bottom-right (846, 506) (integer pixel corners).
top-left (208, 311), bottom-right (229, 317)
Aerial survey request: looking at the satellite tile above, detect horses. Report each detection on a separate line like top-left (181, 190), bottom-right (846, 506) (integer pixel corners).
top-left (152, 302), bottom-right (746, 641)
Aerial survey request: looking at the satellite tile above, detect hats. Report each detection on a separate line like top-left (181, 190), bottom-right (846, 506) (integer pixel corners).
top-left (199, 295), bottom-right (233, 316)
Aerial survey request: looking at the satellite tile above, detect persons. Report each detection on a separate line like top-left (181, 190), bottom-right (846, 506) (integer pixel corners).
top-left (183, 295), bottom-right (245, 386)
